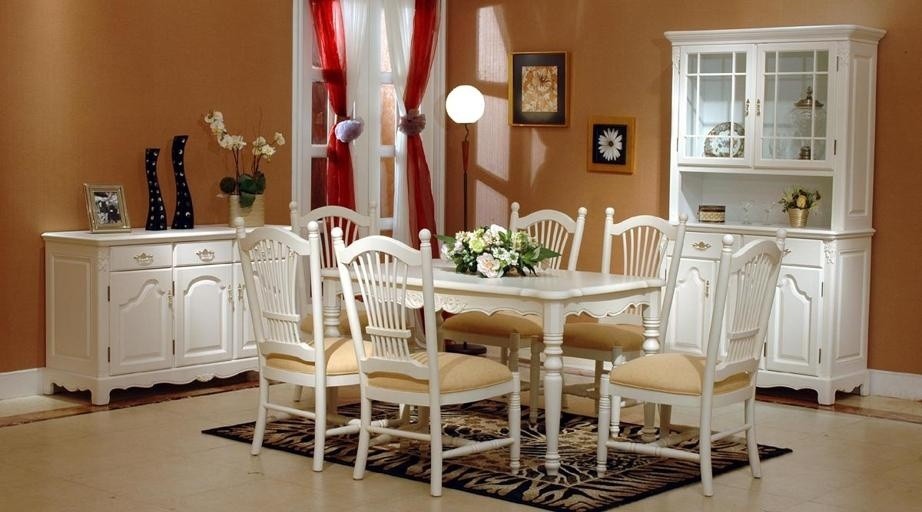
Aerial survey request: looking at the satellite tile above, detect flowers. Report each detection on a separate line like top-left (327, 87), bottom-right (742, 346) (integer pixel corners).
top-left (778, 182), bottom-right (821, 215)
top-left (430, 224), bottom-right (562, 279)
top-left (201, 106), bottom-right (285, 209)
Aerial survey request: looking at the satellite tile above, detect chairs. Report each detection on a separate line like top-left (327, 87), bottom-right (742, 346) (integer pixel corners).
top-left (330, 223), bottom-right (524, 500)
top-left (232, 215), bottom-right (411, 472)
top-left (439, 202), bottom-right (589, 373)
top-left (596, 226), bottom-right (792, 499)
top-left (291, 197), bottom-right (417, 425)
top-left (527, 206), bottom-right (688, 426)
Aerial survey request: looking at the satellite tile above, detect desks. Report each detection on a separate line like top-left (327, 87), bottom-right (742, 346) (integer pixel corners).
top-left (320, 257), bottom-right (670, 479)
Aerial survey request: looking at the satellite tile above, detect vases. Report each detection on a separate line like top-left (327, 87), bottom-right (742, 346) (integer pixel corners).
top-left (227, 192), bottom-right (267, 228)
top-left (787, 207), bottom-right (809, 227)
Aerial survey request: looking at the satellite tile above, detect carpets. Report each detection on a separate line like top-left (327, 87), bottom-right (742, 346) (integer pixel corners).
top-left (201, 399), bottom-right (795, 512)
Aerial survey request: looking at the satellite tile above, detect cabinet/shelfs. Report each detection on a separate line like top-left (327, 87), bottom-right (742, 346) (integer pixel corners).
top-left (658, 19), bottom-right (888, 407)
top-left (38, 222), bottom-right (289, 408)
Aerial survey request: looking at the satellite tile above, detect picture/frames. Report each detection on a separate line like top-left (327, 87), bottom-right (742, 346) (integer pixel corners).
top-left (81, 182), bottom-right (132, 233)
top-left (508, 50), bottom-right (575, 130)
top-left (587, 115), bottom-right (636, 175)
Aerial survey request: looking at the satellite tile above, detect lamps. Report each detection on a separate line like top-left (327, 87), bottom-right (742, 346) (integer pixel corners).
top-left (445, 83), bottom-right (487, 233)
